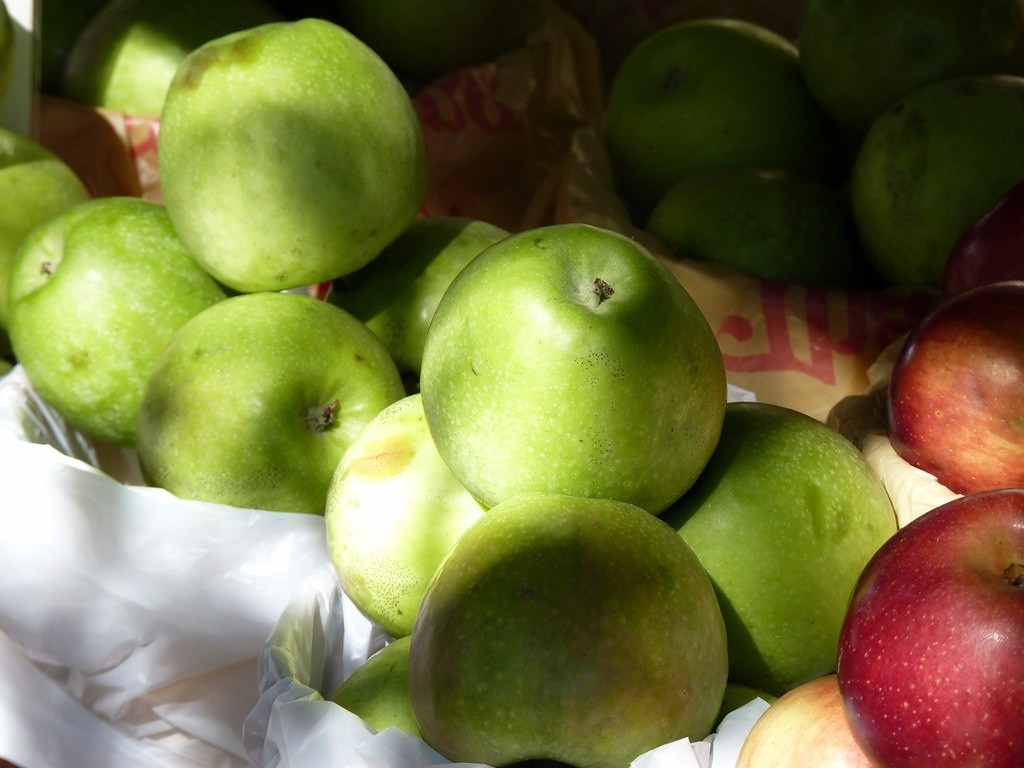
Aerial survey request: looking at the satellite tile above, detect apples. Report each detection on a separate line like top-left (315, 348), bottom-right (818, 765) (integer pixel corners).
top-left (0, 0), bottom-right (1024, 768)
